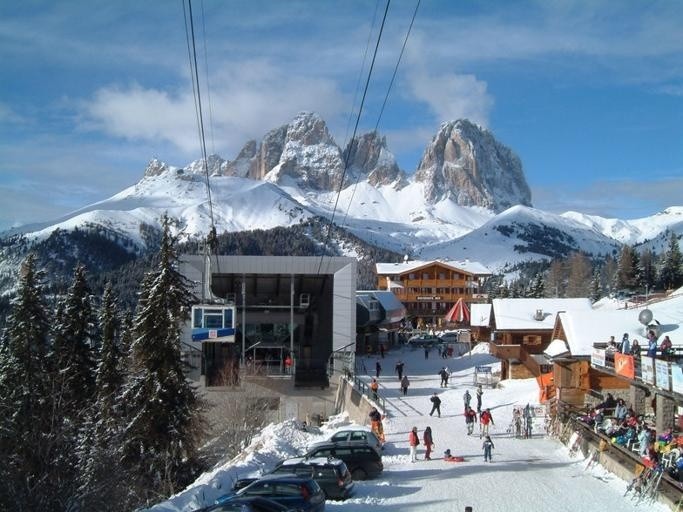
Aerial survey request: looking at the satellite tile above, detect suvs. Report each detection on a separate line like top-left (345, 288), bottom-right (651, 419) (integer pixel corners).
top-left (306, 429), bottom-right (382, 458)
top-left (192, 496), bottom-right (288, 511)
top-left (276, 441), bottom-right (384, 481)
top-left (232, 456), bottom-right (356, 499)
top-left (214, 472), bottom-right (326, 512)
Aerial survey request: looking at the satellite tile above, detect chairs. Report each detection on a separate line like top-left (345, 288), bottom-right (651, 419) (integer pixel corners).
top-left (594, 416), bottom-right (680, 468)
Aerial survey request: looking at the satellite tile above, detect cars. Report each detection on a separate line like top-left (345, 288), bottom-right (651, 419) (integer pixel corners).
top-left (439, 332), bottom-right (459, 343)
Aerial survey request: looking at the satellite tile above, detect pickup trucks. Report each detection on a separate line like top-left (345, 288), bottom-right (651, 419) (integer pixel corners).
top-left (409, 335), bottom-right (440, 347)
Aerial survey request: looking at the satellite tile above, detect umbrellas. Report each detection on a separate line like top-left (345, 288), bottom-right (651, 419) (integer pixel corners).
top-left (443, 296), bottom-right (470, 322)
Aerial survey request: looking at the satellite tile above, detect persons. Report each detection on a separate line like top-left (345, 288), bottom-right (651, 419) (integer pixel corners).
top-left (593, 391), bottom-right (683, 485)
top-left (423, 427), bottom-right (434, 460)
top-left (464, 409), bottom-right (477, 435)
top-left (395, 338), bottom-right (453, 401)
top-left (480, 407), bottom-right (494, 433)
top-left (476, 384), bottom-right (484, 412)
top-left (463, 390), bottom-right (471, 412)
top-left (608, 327), bottom-right (673, 359)
top-left (371, 379), bottom-right (378, 401)
top-left (429, 392), bottom-right (441, 418)
top-left (409, 427), bottom-right (419, 462)
top-left (283, 354), bottom-right (292, 374)
top-left (369, 407), bottom-right (386, 443)
top-left (375, 358), bottom-right (383, 376)
top-left (481, 435), bottom-right (495, 463)
